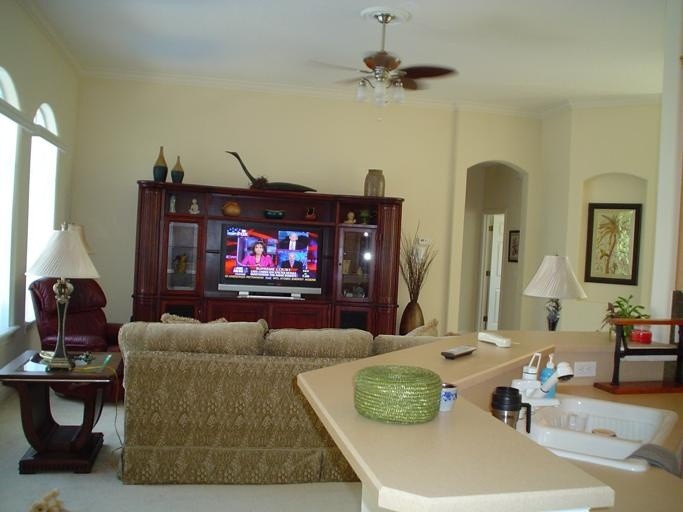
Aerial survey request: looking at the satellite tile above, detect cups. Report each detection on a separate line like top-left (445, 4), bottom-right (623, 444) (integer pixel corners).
top-left (439, 383), bottom-right (458, 413)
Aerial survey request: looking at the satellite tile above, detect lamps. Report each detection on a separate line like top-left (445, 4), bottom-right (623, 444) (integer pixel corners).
top-left (20, 225), bottom-right (98, 374)
top-left (519, 253), bottom-right (590, 331)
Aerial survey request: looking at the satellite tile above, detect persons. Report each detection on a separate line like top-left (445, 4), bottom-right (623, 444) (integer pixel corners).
top-left (241, 242), bottom-right (275, 270)
top-left (278, 233), bottom-right (307, 251)
top-left (281, 254), bottom-right (303, 275)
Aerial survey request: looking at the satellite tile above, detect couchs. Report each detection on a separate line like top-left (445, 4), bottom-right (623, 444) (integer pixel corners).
top-left (118, 320), bottom-right (448, 489)
top-left (27, 277), bottom-right (124, 406)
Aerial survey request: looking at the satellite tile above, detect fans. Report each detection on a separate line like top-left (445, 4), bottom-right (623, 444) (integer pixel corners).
top-left (310, 6), bottom-right (459, 104)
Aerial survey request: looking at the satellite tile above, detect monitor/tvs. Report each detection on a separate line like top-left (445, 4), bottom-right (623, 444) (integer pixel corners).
top-left (217, 222), bottom-right (324, 300)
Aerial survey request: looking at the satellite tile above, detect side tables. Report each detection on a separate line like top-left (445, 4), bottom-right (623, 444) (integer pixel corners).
top-left (2, 350), bottom-right (122, 474)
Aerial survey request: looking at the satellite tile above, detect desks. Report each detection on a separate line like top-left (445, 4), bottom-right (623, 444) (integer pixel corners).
top-left (294, 328), bottom-right (682, 511)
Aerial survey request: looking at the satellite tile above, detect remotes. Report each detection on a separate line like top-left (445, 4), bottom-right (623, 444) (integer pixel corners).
top-left (440, 345), bottom-right (476, 359)
top-left (478, 331), bottom-right (514, 348)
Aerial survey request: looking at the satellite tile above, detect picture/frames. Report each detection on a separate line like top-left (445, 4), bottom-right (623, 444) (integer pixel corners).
top-left (582, 200), bottom-right (645, 286)
top-left (507, 229), bottom-right (520, 263)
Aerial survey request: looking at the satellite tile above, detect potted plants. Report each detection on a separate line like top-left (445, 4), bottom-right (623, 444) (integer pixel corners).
top-left (399, 222), bottom-right (438, 333)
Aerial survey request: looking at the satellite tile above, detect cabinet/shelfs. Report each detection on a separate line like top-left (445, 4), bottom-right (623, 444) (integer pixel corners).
top-left (133, 179), bottom-right (405, 334)
